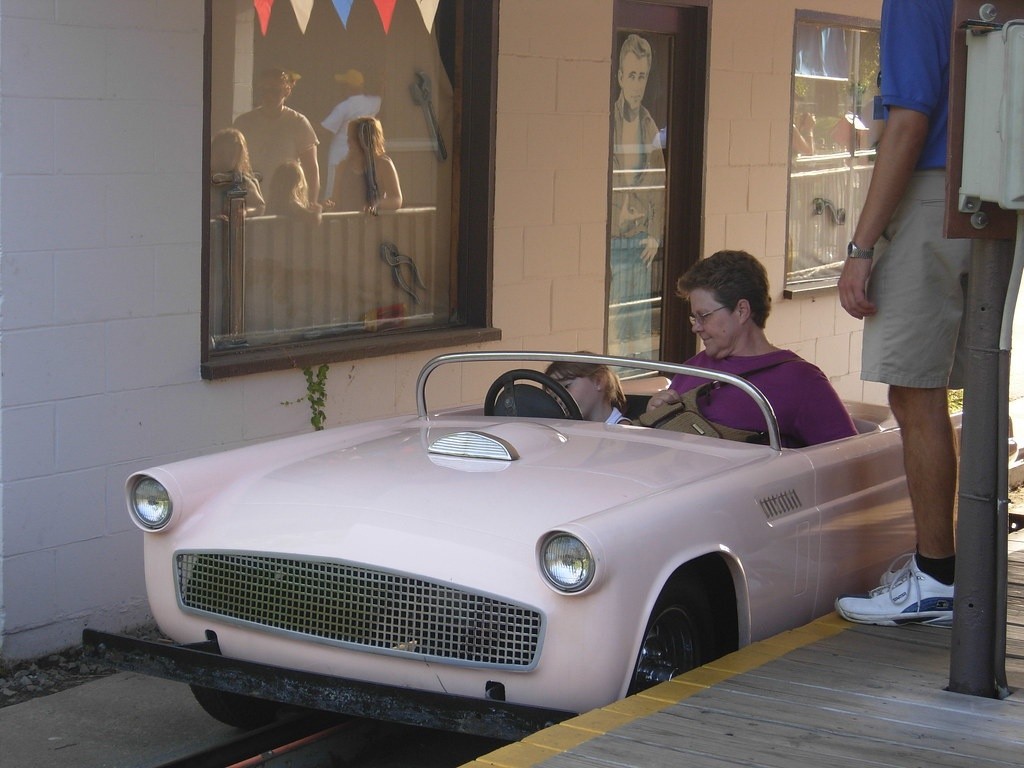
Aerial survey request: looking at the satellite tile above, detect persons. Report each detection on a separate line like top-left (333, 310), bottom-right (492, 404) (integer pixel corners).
top-left (321, 117), bottom-right (403, 216)
top-left (790, 108), bottom-right (816, 172)
top-left (837, 0), bottom-right (978, 627)
top-left (311, 69), bottom-right (385, 202)
top-left (210, 128), bottom-right (267, 222)
top-left (264, 162), bottom-right (323, 228)
top-left (645, 250), bottom-right (859, 450)
top-left (540, 351), bottom-right (634, 427)
top-left (232, 68), bottom-right (323, 214)
top-left (608, 35), bottom-right (667, 374)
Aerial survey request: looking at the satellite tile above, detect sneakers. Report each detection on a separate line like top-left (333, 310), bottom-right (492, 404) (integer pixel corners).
top-left (835, 553), bottom-right (955, 629)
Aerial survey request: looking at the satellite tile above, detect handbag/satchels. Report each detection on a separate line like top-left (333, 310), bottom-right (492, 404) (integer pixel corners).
top-left (631, 358), bottom-right (828, 443)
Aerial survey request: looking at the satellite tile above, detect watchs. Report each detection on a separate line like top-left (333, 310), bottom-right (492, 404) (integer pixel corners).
top-left (847, 242), bottom-right (874, 258)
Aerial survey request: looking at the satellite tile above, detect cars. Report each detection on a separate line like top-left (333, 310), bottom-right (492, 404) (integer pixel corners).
top-left (84, 349), bottom-right (1023, 743)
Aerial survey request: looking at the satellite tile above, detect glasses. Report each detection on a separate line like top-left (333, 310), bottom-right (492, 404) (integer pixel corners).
top-left (688, 305), bottom-right (727, 326)
